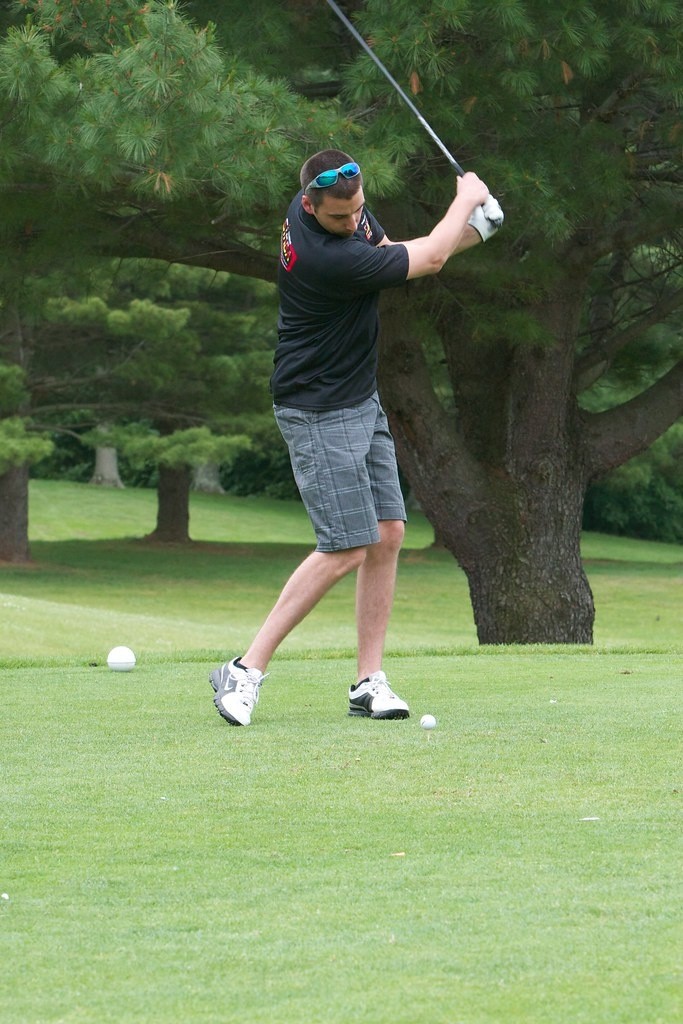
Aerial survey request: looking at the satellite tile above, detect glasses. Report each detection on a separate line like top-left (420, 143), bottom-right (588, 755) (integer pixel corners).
top-left (304, 163), bottom-right (360, 195)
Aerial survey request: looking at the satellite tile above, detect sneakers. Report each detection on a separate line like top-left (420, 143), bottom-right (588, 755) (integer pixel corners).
top-left (347, 671), bottom-right (410, 720)
top-left (208, 657), bottom-right (270, 727)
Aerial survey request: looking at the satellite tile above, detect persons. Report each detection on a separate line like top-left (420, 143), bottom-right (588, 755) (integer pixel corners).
top-left (208, 149), bottom-right (504, 727)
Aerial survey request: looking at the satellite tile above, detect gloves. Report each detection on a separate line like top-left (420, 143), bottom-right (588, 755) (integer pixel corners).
top-left (468, 194), bottom-right (504, 242)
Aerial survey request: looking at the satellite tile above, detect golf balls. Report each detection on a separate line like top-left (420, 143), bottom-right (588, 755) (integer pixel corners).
top-left (421, 714), bottom-right (436, 730)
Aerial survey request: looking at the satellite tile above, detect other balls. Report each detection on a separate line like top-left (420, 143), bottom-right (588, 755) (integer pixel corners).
top-left (107, 644), bottom-right (137, 673)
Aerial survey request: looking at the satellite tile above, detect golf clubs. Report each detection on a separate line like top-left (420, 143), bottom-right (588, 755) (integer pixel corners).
top-left (328, 2), bottom-right (502, 230)
